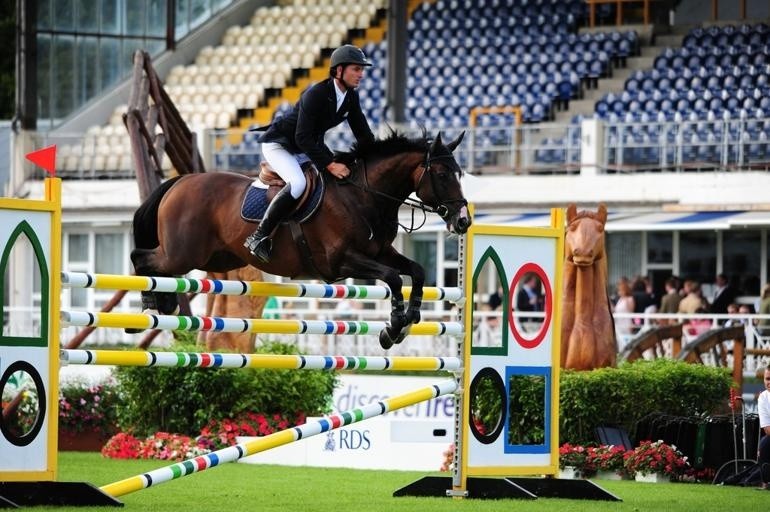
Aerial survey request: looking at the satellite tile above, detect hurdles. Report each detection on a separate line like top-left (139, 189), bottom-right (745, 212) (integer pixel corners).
top-left (0, 178), bottom-right (624, 509)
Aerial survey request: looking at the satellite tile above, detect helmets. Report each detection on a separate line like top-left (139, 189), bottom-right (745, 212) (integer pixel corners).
top-left (331, 44), bottom-right (374, 67)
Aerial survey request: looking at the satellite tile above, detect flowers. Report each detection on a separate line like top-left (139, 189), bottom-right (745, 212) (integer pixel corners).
top-left (558, 439), bottom-right (695, 477)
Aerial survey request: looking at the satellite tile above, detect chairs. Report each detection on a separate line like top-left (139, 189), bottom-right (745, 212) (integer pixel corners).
top-left (50, 2), bottom-right (768, 177)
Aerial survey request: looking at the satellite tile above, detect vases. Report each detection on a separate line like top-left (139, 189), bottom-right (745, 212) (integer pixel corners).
top-left (555, 465), bottom-right (671, 482)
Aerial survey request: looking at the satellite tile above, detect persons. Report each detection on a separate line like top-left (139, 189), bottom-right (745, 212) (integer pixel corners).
top-left (244, 44), bottom-right (376, 264)
top-left (517, 275), bottom-right (541, 321)
top-left (756, 364), bottom-right (770, 489)
top-left (604, 272), bottom-right (766, 355)
top-left (486, 294), bottom-right (502, 325)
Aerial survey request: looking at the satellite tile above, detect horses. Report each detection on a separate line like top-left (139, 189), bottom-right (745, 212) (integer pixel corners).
top-left (125, 125), bottom-right (472, 350)
top-left (560, 202), bottom-right (618, 371)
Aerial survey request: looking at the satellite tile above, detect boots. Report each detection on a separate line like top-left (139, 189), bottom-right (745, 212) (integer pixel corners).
top-left (243, 183), bottom-right (296, 264)
